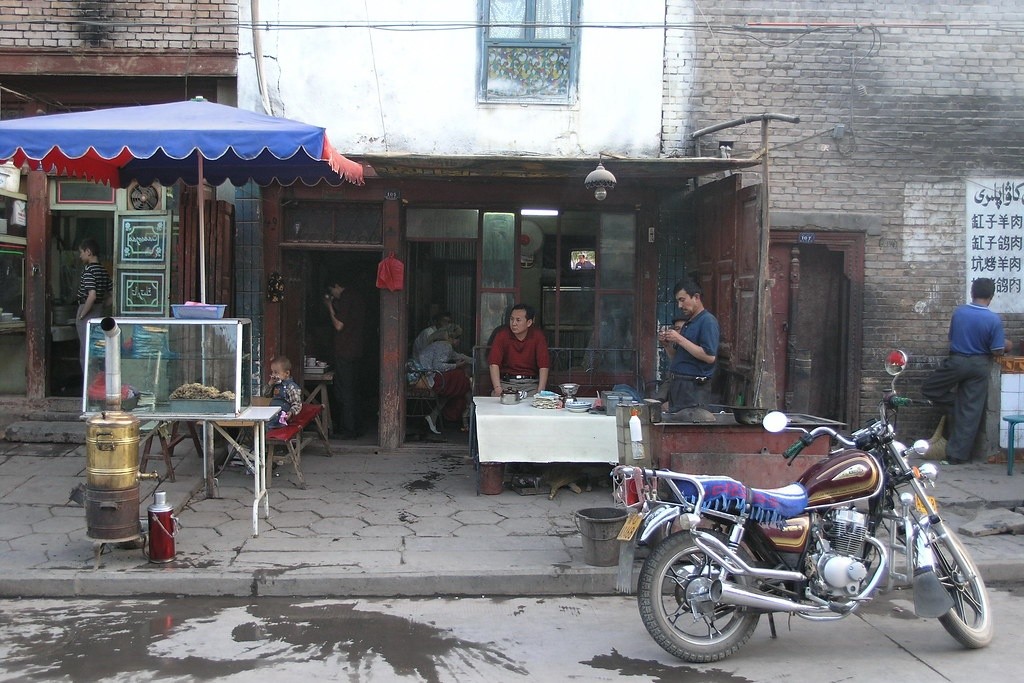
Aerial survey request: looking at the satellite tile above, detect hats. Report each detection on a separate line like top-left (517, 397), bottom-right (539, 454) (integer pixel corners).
top-left (672, 315), bottom-right (689, 324)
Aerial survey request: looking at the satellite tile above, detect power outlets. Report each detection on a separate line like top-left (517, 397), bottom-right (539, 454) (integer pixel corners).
top-left (648, 227), bottom-right (655, 242)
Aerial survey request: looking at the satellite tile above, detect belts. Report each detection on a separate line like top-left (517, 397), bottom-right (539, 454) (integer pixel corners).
top-left (78, 301), bottom-right (102, 304)
top-left (669, 373), bottom-right (695, 381)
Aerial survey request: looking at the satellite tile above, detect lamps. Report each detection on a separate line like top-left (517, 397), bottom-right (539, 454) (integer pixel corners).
top-left (580, 156), bottom-right (619, 202)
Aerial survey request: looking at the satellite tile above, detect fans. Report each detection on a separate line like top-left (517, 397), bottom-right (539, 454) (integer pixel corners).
top-left (520, 220), bottom-right (545, 269)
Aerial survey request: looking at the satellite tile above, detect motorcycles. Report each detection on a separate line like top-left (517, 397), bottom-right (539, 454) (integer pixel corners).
top-left (614, 350), bottom-right (997, 662)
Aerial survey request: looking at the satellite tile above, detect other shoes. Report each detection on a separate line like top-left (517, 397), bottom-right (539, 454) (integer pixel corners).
top-left (331, 430), bottom-right (356, 440)
top-left (461, 424), bottom-right (469, 432)
top-left (948, 458), bottom-right (958, 465)
top-left (507, 463), bottom-right (536, 474)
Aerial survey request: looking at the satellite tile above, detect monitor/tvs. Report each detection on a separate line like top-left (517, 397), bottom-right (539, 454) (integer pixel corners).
top-left (569, 249), bottom-right (596, 271)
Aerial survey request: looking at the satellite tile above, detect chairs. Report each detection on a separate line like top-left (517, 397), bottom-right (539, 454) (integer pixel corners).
top-left (405, 359), bottom-right (445, 429)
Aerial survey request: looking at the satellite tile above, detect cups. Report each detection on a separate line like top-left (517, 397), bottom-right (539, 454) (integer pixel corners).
top-left (304, 355), bottom-right (327, 367)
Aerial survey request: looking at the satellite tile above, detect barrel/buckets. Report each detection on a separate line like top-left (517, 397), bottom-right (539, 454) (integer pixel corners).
top-left (575, 507), bottom-right (630, 567)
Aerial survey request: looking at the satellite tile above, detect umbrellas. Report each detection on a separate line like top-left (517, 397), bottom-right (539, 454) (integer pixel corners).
top-left (0, 96), bottom-right (366, 480)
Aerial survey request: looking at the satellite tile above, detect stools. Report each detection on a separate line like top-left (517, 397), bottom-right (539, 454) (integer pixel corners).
top-left (1003, 414), bottom-right (1024, 475)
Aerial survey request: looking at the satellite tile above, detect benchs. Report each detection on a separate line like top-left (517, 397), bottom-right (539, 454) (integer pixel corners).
top-left (140, 419), bottom-right (200, 486)
top-left (267, 402), bottom-right (332, 491)
top-left (468, 346), bottom-right (645, 457)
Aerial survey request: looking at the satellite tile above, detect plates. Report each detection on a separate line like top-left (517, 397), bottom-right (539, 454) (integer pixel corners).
top-left (566, 401), bottom-right (592, 412)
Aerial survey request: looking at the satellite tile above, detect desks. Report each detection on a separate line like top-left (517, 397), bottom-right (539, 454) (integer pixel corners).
top-left (299, 362), bottom-right (339, 439)
top-left (80, 406), bottom-right (282, 491)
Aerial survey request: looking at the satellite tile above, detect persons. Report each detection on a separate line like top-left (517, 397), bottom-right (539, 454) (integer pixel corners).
top-left (922, 278), bottom-right (1012, 464)
top-left (76, 238), bottom-right (113, 372)
top-left (487, 303), bottom-right (551, 397)
top-left (324, 270), bottom-right (364, 439)
top-left (652, 279), bottom-right (721, 412)
top-left (262, 357), bottom-right (302, 434)
top-left (413, 313), bottom-right (474, 435)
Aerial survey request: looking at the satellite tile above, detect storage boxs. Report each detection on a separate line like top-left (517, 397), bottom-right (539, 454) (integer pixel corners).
top-left (304, 365), bottom-right (330, 374)
top-left (170, 304), bottom-right (228, 319)
top-left (169, 399), bottom-right (236, 413)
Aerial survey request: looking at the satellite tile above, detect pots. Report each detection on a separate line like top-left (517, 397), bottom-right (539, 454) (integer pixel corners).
top-left (85, 411), bottom-right (140, 490)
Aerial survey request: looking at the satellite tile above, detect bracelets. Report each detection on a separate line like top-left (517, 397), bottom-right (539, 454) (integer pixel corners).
top-left (492, 385), bottom-right (502, 390)
top-left (79, 313), bottom-right (83, 319)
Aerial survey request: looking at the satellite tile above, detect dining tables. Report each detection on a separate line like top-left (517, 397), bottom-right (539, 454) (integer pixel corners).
top-left (473, 396), bottom-right (619, 497)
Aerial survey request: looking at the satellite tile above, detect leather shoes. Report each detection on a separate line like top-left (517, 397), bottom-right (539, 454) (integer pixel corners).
top-left (423, 415), bottom-right (441, 435)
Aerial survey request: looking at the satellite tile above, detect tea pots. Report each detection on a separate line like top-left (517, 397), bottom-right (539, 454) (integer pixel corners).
top-left (499, 387), bottom-right (520, 405)
top-left (732, 407), bottom-right (769, 425)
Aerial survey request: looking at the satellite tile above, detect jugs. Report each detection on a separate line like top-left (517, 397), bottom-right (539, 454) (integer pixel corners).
top-left (147, 491), bottom-right (183, 563)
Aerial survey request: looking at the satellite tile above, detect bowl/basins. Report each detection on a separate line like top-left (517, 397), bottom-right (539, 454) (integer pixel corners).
top-left (0, 307), bottom-right (22, 322)
